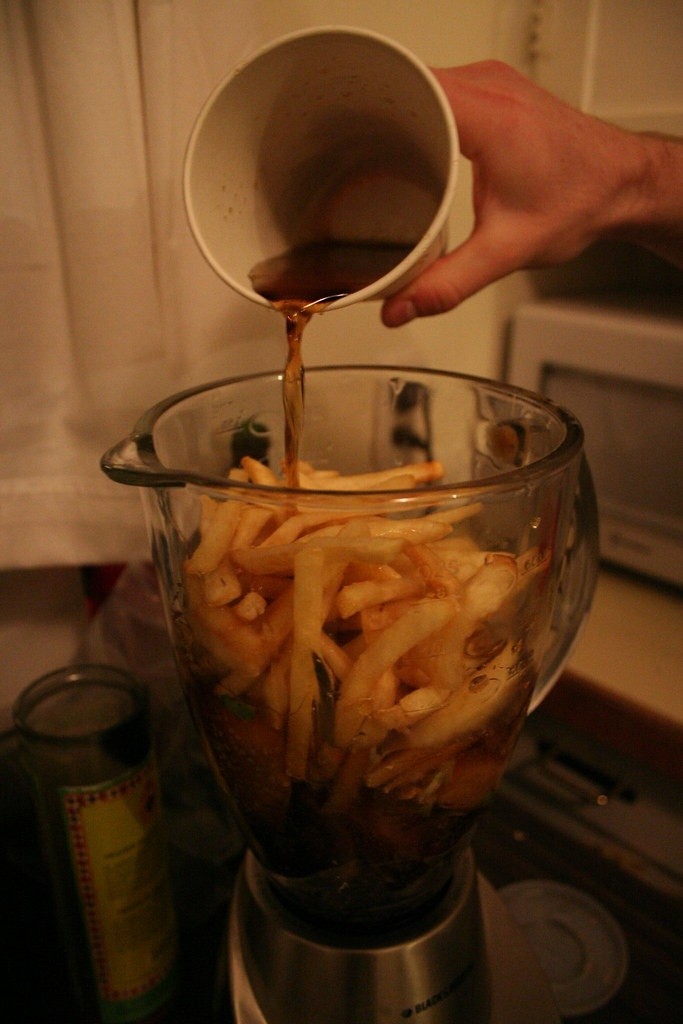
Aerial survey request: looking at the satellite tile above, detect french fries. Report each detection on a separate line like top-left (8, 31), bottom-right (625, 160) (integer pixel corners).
top-left (184, 458), bottom-right (555, 823)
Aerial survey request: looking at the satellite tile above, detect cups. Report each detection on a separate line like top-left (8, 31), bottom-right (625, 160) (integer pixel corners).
top-left (181, 25), bottom-right (461, 316)
top-left (12, 661), bottom-right (183, 1024)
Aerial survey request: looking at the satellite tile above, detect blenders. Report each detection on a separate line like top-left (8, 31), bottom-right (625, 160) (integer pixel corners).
top-left (101, 363), bottom-right (602, 1024)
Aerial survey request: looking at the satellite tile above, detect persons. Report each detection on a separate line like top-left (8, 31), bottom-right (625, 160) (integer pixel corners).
top-left (380, 58), bottom-right (683, 330)
